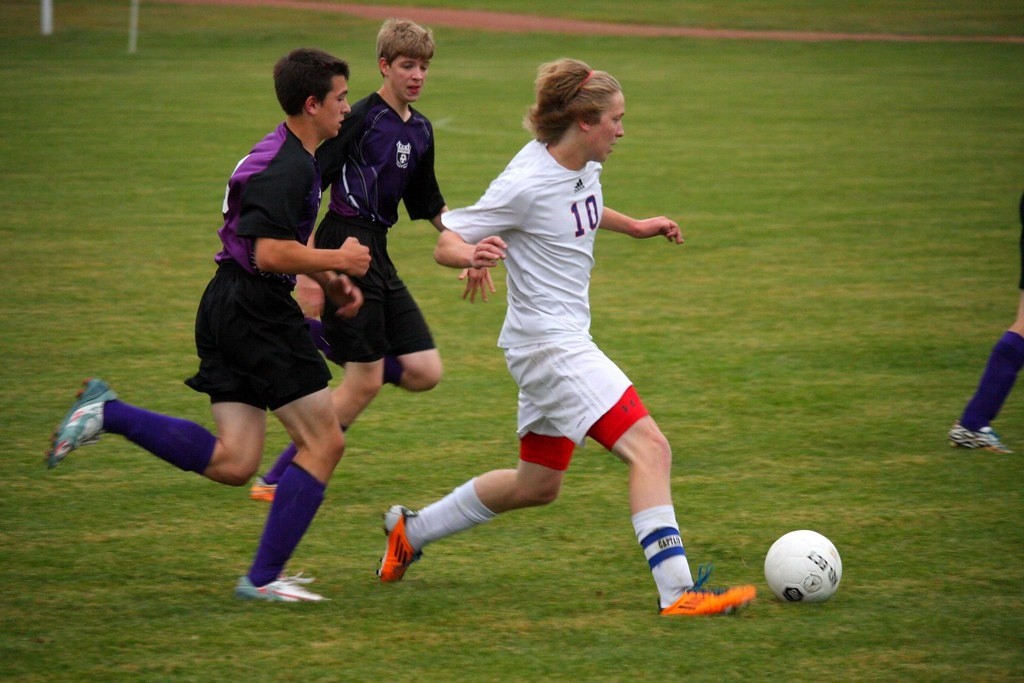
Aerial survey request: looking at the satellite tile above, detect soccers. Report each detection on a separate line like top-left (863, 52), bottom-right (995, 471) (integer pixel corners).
top-left (764, 529), bottom-right (842, 603)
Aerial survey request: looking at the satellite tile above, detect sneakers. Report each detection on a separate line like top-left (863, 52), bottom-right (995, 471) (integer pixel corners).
top-left (234, 574), bottom-right (328, 603)
top-left (948, 419), bottom-right (1014, 454)
top-left (44, 376), bottom-right (117, 471)
top-left (658, 582), bottom-right (757, 619)
top-left (250, 476), bottom-right (277, 502)
top-left (378, 504), bottom-right (422, 584)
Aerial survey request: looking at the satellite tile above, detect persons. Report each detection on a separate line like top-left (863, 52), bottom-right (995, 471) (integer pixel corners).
top-left (377, 55), bottom-right (757, 621)
top-left (947, 191), bottom-right (1024, 455)
top-left (251, 19), bottom-right (497, 503)
top-left (44, 46), bottom-right (371, 605)
